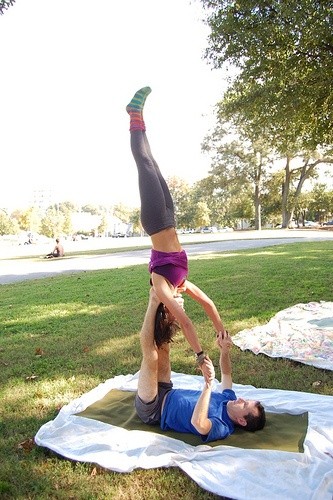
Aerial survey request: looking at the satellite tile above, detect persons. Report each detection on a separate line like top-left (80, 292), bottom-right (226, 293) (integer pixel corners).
top-left (135, 287), bottom-right (267, 444)
top-left (52, 239), bottom-right (64, 257)
top-left (125, 86), bottom-right (232, 388)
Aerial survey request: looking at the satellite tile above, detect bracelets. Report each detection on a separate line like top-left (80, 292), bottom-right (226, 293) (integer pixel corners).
top-left (216, 335), bottom-right (224, 339)
top-left (195, 351), bottom-right (206, 364)
